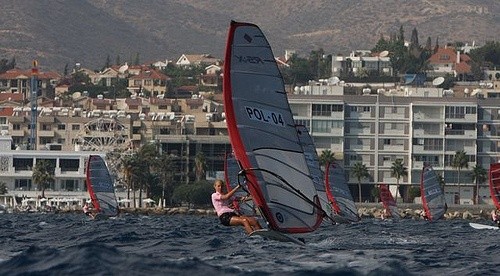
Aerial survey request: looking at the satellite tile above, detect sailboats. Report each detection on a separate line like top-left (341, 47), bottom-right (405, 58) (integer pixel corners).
top-left (379, 183), bottom-right (402, 224)
top-left (223, 126), bottom-right (340, 234)
top-left (327, 162), bottom-right (361, 225)
top-left (223, 19), bottom-right (326, 244)
top-left (86, 155), bottom-right (121, 221)
top-left (468, 163), bottom-right (500, 230)
top-left (420, 165), bottom-right (447, 226)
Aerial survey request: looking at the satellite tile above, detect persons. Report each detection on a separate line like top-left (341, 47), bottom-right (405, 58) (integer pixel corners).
top-left (378, 208), bottom-right (385, 221)
top-left (491, 209), bottom-right (500, 228)
top-left (417, 208), bottom-right (429, 222)
top-left (210, 179), bottom-right (261, 233)
top-left (82, 202), bottom-right (95, 219)
